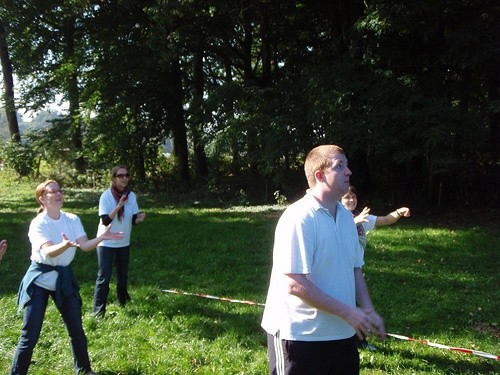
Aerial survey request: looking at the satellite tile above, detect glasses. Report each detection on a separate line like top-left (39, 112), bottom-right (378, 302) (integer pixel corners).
top-left (115, 173), bottom-right (129, 178)
top-left (43, 189), bottom-right (62, 195)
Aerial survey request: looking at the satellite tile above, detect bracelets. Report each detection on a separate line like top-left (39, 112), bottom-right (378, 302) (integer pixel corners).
top-left (396, 209), bottom-right (401, 217)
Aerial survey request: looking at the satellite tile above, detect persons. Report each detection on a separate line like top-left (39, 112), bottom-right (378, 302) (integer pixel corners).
top-left (10, 180), bottom-right (125, 375)
top-left (261, 145), bottom-right (385, 375)
top-left (339, 185), bottom-right (411, 352)
top-left (0, 239), bottom-right (8, 259)
top-left (92, 165), bottom-right (146, 322)
